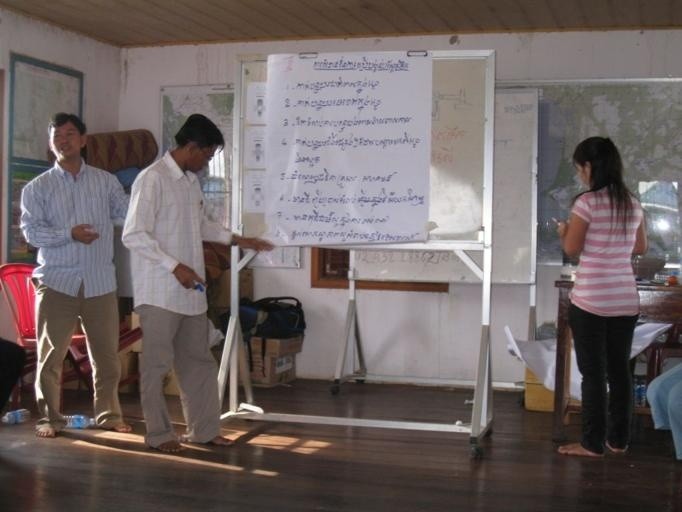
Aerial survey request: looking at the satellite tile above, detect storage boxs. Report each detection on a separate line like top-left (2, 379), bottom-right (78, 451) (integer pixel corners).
top-left (56, 311), bottom-right (182, 396)
top-left (249, 334), bottom-right (302, 387)
top-left (523, 365), bottom-right (557, 416)
top-left (204, 266), bottom-right (253, 308)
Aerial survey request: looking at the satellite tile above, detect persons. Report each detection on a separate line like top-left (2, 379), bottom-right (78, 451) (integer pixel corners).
top-left (122, 113), bottom-right (274, 455)
top-left (556, 132), bottom-right (648, 458)
top-left (0, 335), bottom-right (26, 411)
top-left (646, 361), bottom-right (682, 463)
top-left (20, 113), bottom-right (132, 436)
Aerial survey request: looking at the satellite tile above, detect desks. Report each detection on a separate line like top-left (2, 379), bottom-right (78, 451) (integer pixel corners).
top-left (548, 280), bottom-right (682, 447)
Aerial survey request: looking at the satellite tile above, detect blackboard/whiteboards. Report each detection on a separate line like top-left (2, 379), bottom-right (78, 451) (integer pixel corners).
top-left (230, 48), bottom-right (497, 251)
top-left (347, 87), bottom-right (539, 285)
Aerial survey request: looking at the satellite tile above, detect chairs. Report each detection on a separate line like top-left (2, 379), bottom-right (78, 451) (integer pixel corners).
top-left (0, 262), bottom-right (93, 411)
top-left (7, 320), bottom-right (142, 410)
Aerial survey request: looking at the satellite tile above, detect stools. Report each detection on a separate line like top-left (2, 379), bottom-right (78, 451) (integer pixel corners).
top-left (627, 341), bottom-right (682, 430)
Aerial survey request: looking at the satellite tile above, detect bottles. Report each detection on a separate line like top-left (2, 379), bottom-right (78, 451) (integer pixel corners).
top-left (61, 414), bottom-right (97, 430)
top-left (1, 408), bottom-right (33, 426)
top-left (654, 270), bottom-right (682, 284)
top-left (634, 376), bottom-right (648, 408)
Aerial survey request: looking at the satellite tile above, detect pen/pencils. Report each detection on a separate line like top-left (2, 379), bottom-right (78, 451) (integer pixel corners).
top-left (193, 280), bottom-right (204, 292)
top-left (551, 217), bottom-right (561, 226)
